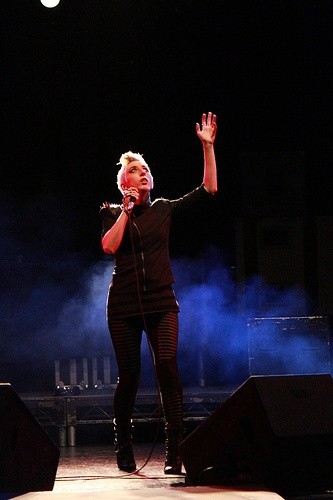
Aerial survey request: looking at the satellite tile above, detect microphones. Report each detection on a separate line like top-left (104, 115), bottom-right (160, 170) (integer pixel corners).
top-left (127, 197), bottom-right (136, 213)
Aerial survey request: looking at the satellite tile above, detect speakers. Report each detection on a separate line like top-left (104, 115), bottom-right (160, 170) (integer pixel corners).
top-left (0, 382), bottom-right (61, 492)
top-left (177, 373), bottom-right (333, 487)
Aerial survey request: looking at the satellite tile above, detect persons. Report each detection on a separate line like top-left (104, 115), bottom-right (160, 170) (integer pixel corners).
top-left (100, 111), bottom-right (219, 473)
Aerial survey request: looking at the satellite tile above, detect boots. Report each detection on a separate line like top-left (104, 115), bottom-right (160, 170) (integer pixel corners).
top-left (112, 417), bottom-right (136, 472)
top-left (163, 423), bottom-right (186, 474)
